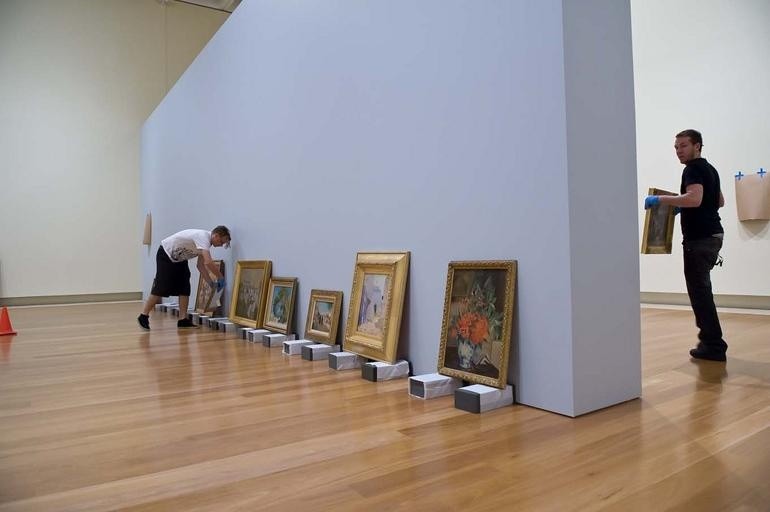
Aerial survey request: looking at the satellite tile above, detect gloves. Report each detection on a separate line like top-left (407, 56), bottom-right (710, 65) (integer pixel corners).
top-left (645, 195), bottom-right (659, 209)
top-left (215, 278), bottom-right (224, 293)
top-left (674, 207), bottom-right (680, 216)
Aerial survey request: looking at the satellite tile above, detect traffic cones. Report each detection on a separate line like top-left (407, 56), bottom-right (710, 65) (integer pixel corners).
top-left (0, 306), bottom-right (19, 336)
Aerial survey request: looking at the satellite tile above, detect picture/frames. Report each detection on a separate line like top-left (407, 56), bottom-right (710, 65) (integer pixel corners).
top-left (262, 276), bottom-right (297, 336)
top-left (304, 289), bottom-right (343, 346)
top-left (640, 189), bottom-right (679, 255)
top-left (341, 250), bottom-right (410, 365)
top-left (228, 258), bottom-right (274, 330)
top-left (435, 259), bottom-right (518, 390)
top-left (194, 258), bottom-right (224, 319)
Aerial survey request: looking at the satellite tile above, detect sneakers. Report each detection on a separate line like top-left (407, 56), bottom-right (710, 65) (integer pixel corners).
top-left (178, 318), bottom-right (198, 328)
top-left (138, 314), bottom-right (150, 330)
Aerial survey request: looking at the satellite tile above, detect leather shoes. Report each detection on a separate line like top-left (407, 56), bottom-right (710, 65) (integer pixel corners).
top-left (690, 349), bottom-right (726, 361)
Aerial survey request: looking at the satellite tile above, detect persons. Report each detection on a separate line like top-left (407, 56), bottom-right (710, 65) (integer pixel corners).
top-left (136, 225), bottom-right (232, 331)
top-left (644, 128), bottom-right (728, 363)
